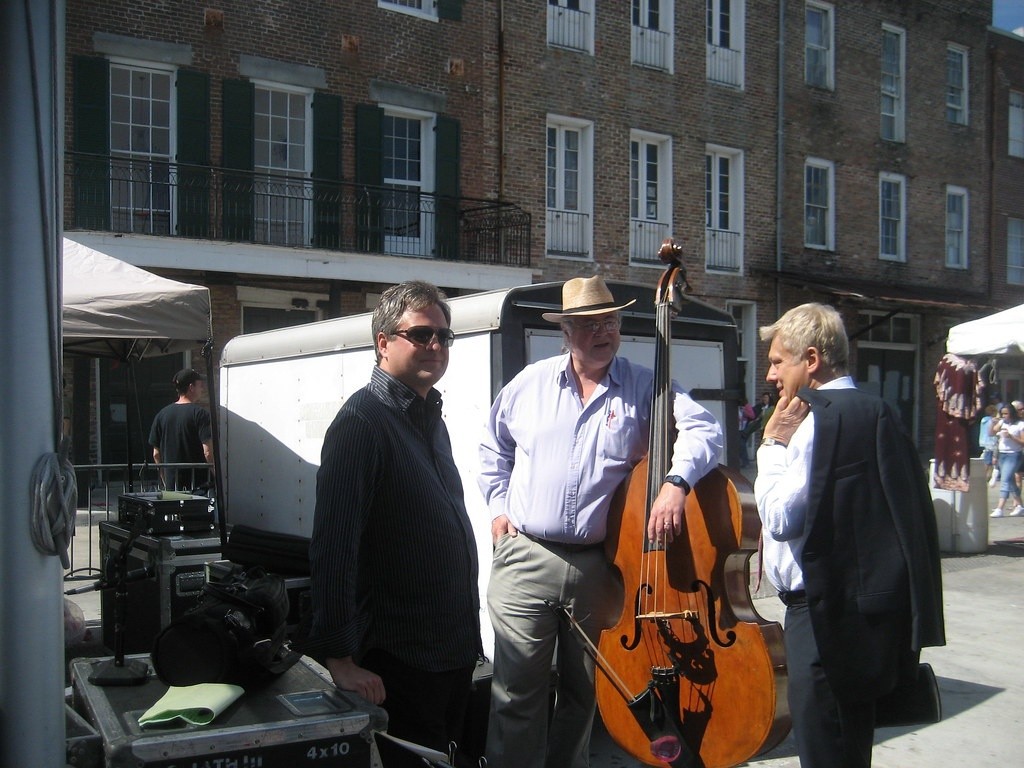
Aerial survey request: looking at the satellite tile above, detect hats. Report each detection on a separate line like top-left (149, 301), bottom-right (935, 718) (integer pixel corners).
top-left (542, 275), bottom-right (636, 323)
top-left (173, 368), bottom-right (207, 385)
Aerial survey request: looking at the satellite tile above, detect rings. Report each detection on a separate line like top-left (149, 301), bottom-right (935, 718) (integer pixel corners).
top-left (663, 522), bottom-right (672, 525)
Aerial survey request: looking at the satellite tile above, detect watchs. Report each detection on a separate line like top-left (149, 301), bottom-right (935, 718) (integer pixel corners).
top-left (662, 475), bottom-right (691, 495)
top-left (759, 438), bottom-right (787, 449)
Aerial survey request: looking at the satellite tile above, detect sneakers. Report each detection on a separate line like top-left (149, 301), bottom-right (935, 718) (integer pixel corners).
top-left (990, 508), bottom-right (1003, 518)
top-left (1010, 507), bottom-right (1024, 516)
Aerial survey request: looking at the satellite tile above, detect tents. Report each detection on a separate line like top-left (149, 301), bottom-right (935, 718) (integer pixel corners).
top-left (935, 304), bottom-right (1024, 489)
top-left (61, 236), bottom-right (229, 571)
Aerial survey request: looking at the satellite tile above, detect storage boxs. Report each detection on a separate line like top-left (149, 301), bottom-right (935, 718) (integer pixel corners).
top-left (117, 491), bottom-right (225, 538)
top-left (72, 652), bottom-right (388, 768)
top-left (100, 521), bottom-right (265, 652)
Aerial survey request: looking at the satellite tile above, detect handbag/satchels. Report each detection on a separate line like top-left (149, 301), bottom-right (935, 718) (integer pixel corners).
top-left (150, 574), bottom-right (306, 686)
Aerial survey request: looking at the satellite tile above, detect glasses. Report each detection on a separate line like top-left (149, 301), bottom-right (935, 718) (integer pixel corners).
top-left (389, 326), bottom-right (455, 347)
top-left (568, 318), bottom-right (621, 334)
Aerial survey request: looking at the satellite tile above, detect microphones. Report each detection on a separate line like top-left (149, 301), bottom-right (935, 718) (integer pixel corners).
top-left (66, 567), bottom-right (155, 595)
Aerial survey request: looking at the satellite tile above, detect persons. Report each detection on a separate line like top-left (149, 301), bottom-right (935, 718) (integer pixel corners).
top-left (149, 368), bottom-right (215, 504)
top-left (753, 303), bottom-right (946, 768)
top-left (470, 275), bottom-right (722, 768)
top-left (978, 399), bottom-right (1024, 517)
top-left (739, 393), bottom-right (776, 467)
top-left (309, 282), bottom-right (483, 768)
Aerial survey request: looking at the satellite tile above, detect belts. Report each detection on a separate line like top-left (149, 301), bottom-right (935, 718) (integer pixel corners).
top-left (517, 531), bottom-right (605, 551)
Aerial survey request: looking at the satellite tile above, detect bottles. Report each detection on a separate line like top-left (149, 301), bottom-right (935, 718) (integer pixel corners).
top-left (993, 418), bottom-right (1003, 432)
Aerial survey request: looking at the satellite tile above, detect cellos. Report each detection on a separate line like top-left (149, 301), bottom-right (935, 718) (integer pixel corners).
top-left (596, 237), bottom-right (796, 767)
